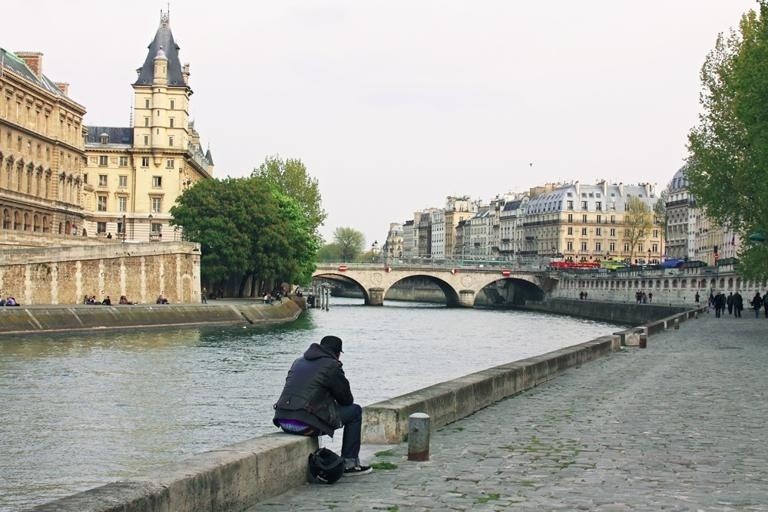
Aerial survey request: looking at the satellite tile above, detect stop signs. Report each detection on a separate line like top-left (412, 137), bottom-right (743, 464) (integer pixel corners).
top-left (338, 266), bottom-right (346, 272)
top-left (502, 269), bottom-right (511, 276)
top-left (715, 253), bottom-right (718, 259)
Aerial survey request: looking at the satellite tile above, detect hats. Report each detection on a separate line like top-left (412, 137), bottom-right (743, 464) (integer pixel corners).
top-left (319, 335), bottom-right (345, 353)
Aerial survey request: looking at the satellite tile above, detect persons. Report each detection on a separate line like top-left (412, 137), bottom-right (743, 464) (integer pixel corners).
top-left (708, 289), bottom-right (768, 319)
top-left (1, 295), bottom-right (18, 305)
top-left (201, 287), bottom-right (208, 304)
top-left (156, 294), bottom-right (170, 305)
top-left (579, 290), bottom-right (588, 299)
top-left (272, 335), bottom-right (374, 478)
top-left (635, 289), bottom-right (653, 305)
top-left (263, 285), bottom-right (302, 305)
top-left (82, 293), bottom-right (133, 305)
top-left (695, 291), bottom-right (700, 303)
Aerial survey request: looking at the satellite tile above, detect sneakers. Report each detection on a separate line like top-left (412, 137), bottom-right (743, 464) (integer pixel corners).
top-left (343, 459), bottom-right (373, 477)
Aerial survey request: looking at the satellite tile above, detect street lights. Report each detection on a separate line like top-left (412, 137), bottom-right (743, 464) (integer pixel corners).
top-left (148, 214), bottom-right (153, 243)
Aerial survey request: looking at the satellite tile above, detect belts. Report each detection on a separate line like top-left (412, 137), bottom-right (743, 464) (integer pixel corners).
top-left (278, 424), bottom-right (312, 434)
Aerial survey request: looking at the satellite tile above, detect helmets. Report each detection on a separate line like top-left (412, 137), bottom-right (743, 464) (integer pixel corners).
top-left (308, 447), bottom-right (344, 484)
